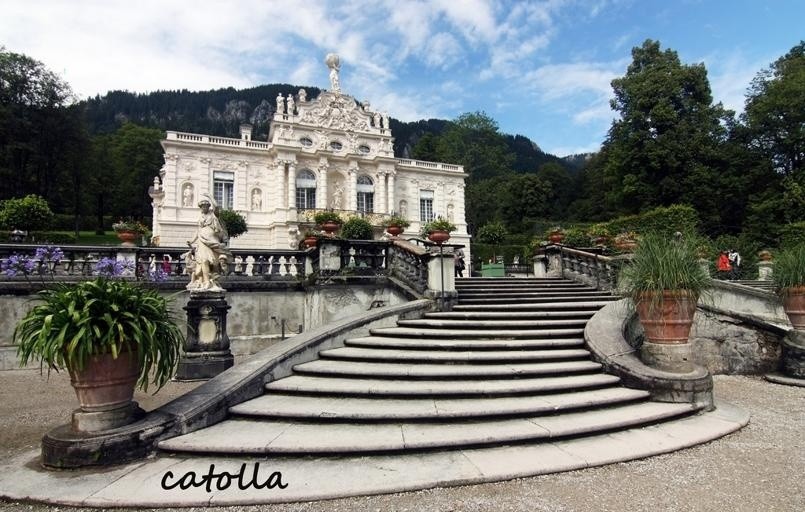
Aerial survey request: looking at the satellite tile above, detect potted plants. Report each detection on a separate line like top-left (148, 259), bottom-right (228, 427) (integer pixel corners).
top-left (296, 207), bottom-right (457, 242)
top-left (113, 219), bottom-right (154, 247)
top-left (548, 218), bottom-right (805, 344)
top-left (477, 222), bottom-right (508, 277)
top-left (12, 273), bottom-right (196, 413)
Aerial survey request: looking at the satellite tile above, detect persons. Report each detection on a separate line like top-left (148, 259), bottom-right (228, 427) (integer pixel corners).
top-left (717, 250), bottom-right (731, 279)
top-left (329, 64), bottom-right (341, 91)
top-left (287, 93), bottom-right (294, 115)
top-left (373, 110), bottom-right (381, 128)
top-left (383, 111), bottom-right (389, 128)
top-left (276, 92), bottom-right (285, 113)
top-left (456, 248), bottom-right (465, 277)
top-left (362, 98), bottom-right (370, 112)
top-left (181, 199), bottom-right (223, 290)
top-left (184, 185), bottom-right (192, 205)
top-left (448, 205), bottom-right (453, 222)
top-left (400, 201), bottom-right (407, 218)
top-left (728, 248), bottom-right (741, 278)
top-left (253, 190), bottom-right (260, 208)
top-left (332, 182), bottom-right (345, 209)
top-left (163, 255), bottom-right (170, 274)
